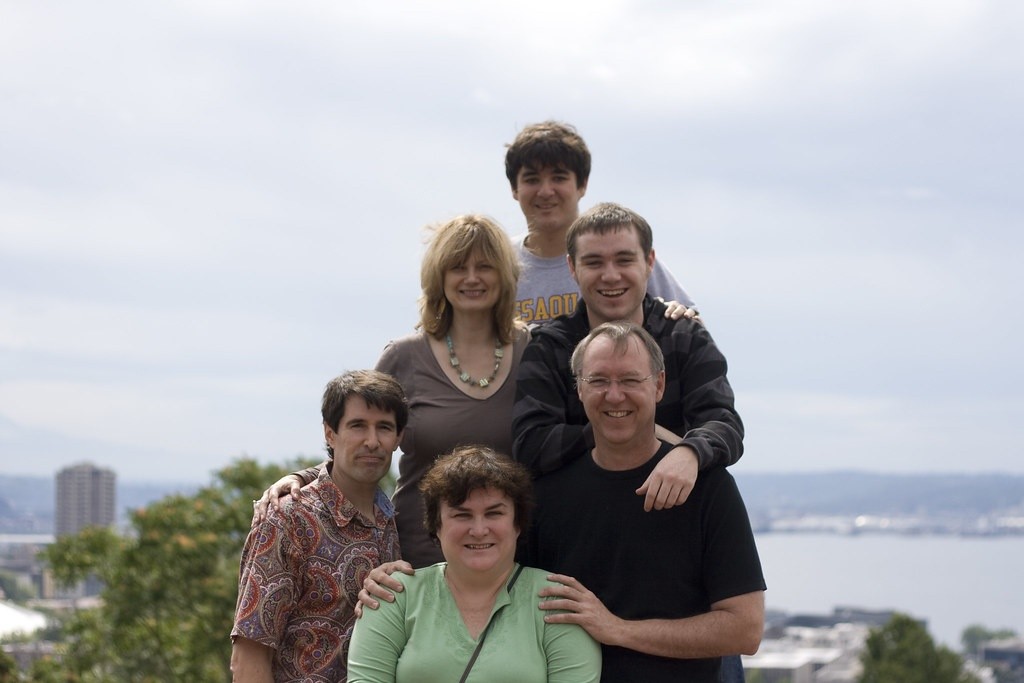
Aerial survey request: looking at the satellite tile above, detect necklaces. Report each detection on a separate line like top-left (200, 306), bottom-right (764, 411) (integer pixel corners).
top-left (445, 333), bottom-right (504, 388)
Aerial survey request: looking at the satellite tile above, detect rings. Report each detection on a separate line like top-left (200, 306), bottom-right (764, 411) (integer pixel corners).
top-left (252, 500), bottom-right (257, 504)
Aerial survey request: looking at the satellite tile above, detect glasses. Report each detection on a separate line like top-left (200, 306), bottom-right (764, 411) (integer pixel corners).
top-left (579, 371), bottom-right (655, 394)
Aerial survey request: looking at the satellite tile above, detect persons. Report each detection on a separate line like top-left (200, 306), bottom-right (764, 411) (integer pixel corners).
top-left (346, 443), bottom-right (602, 683)
top-left (251, 213), bottom-right (534, 527)
top-left (504, 122), bottom-right (698, 319)
top-left (228, 369), bottom-right (402, 683)
top-left (509, 202), bottom-right (746, 513)
top-left (355, 321), bottom-right (767, 683)
